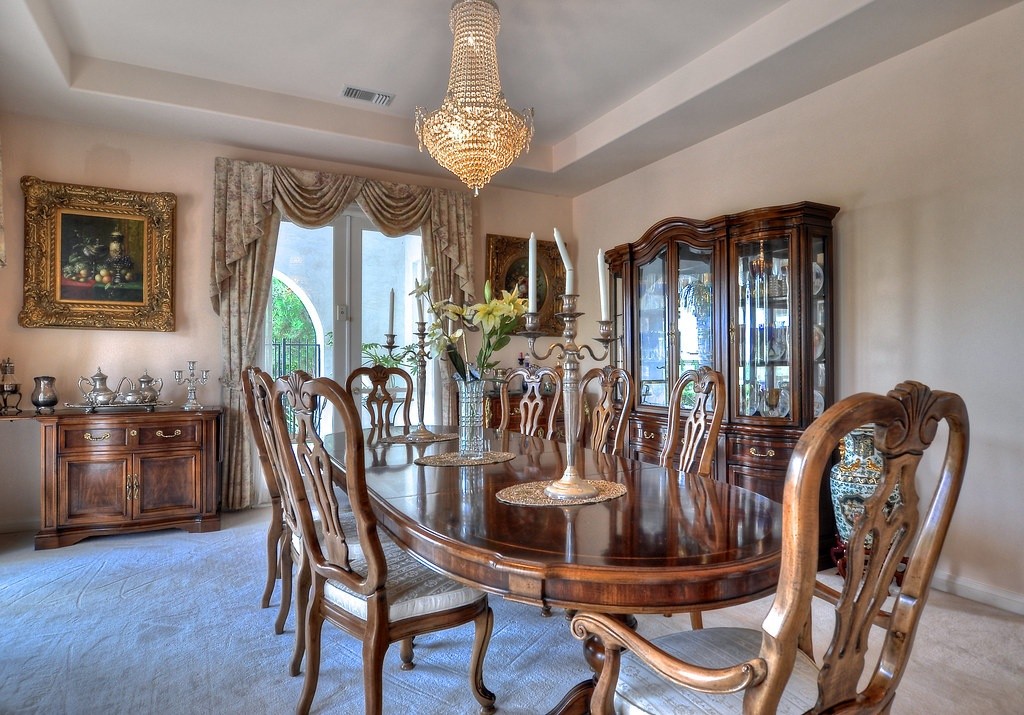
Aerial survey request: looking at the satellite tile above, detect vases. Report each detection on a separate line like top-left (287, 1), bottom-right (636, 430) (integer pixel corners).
top-left (455, 379), bottom-right (487, 454)
top-left (829, 426), bottom-right (904, 548)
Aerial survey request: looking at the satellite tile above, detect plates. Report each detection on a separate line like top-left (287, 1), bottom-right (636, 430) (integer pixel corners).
top-left (740, 384), bottom-right (791, 418)
top-left (813, 390), bottom-right (824, 418)
top-left (812, 261), bottom-right (824, 295)
top-left (758, 334), bottom-right (786, 360)
top-left (813, 326), bottom-right (825, 360)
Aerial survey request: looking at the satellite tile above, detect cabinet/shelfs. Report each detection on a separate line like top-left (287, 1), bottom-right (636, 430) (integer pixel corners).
top-left (34, 405), bottom-right (224, 552)
top-left (605, 200), bottom-right (842, 569)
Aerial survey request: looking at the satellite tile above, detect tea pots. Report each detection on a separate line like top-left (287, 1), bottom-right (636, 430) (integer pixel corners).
top-left (127, 368), bottom-right (163, 404)
top-left (78, 366), bottom-right (126, 406)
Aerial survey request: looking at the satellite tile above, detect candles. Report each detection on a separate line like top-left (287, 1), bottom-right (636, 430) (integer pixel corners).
top-left (448, 293), bottom-right (455, 336)
top-left (552, 226), bottom-right (574, 295)
top-left (528, 232), bottom-right (537, 313)
top-left (414, 278), bottom-right (424, 322)
top-left (597, 248), bottom-right (610, 321)
top-left (388, 287), bottom-right (395, 334)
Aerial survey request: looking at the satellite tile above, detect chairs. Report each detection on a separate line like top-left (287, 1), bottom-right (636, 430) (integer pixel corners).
top-left (242, 365), bottom-right (338, 608)
top-left (271, 371), bottom-right (494, 715)
top-left (577, 364), bottom-right (634, 456)
top-left (346, 365), bottom-right (413, 426)
top-left (570, 380), bottom-right (970, 715)
top-left (658, 366), bottom-right (726, 631)
top-left (496, 366), bottom-right (563, 440)
top-left (254, 372), bottom-right (415, 677)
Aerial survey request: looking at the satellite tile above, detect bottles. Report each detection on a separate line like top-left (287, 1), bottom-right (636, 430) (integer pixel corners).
top-left (830, 426), bottom-right (907, 546)
top-left (522, 354), bottom-right (534, 393)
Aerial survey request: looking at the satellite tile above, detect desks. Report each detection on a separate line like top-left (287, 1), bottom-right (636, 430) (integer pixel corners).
top-left (322, 426), bottom-right (783, 715)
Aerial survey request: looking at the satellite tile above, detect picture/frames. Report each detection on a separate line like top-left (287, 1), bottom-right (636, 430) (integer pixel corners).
top-left (18, 175), bottom-right (177, 332)
top-left (485, 233), bottom-right (567, 337)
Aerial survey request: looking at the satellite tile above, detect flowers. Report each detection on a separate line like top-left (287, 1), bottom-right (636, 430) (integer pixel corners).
top-left (410, 279), bottom-right (528, 379)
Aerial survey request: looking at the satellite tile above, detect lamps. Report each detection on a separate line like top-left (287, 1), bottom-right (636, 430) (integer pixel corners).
top-left (413, 0), bottom-right (535, 199)
